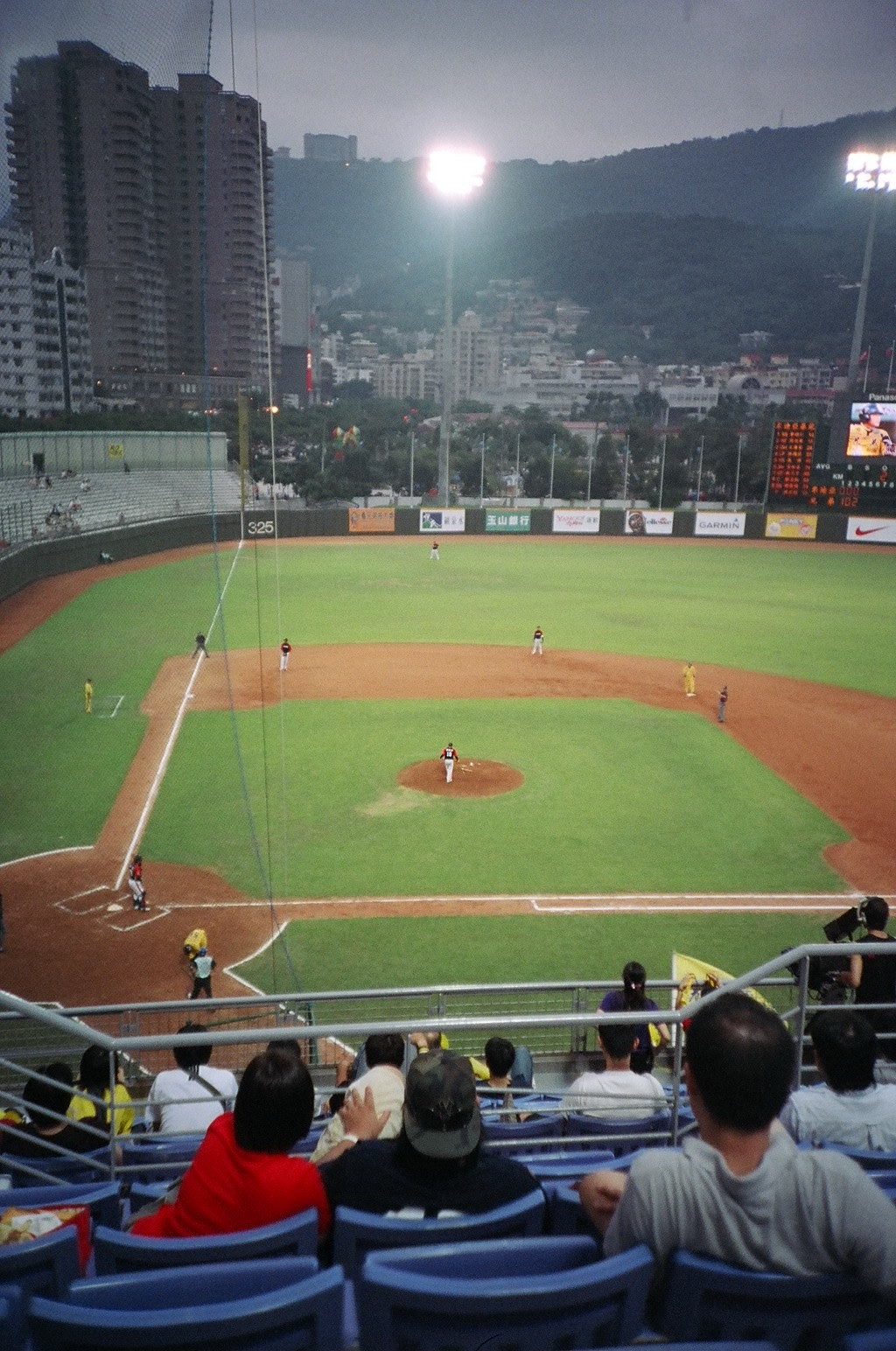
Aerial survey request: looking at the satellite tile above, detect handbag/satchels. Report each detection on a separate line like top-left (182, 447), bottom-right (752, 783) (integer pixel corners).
top-left (0, 1202), bottom-right (91, 1281)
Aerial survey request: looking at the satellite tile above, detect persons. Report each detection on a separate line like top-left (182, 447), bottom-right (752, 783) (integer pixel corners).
top-left (60, 468), bottom-right (76, 479)
top-left (279, 638), bottom-right (291, 672)
top-left (430, 540), bottom-right (440, 561)
top-left (129, 854), bottom-right (151, 911)
top-left (718, 685), bottom-right (728, 724)
top-left (27, 476), bottom-right (52, 490)
top-left (0, 894), bottom-right (896, 1306)
top-left (191, 631), bottom-right (210, 659)
top-left (439, 742), bottom-right (460, 784)
top-left (32, 495), bottom-right (83, 542)
top-left (84, 677), bottom-right (93, 713)
top-left (123, 460), bottom-right (131, 474)
top-left (847, 403), bottom-right (891, 455)
top-left (80, 479), bottom-right (91, 492)
top-left (532, 626), bottom-right (544, 655)
top-left (682, 661), bottom-right (698, 696)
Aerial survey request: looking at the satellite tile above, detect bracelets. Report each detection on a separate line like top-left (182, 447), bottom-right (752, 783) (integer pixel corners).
top-left (336, 1134), bottom-right (359, 1145)
top-left (418, 1046), bottom-right (429, 1055)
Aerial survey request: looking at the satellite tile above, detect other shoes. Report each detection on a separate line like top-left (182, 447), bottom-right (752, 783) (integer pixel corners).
top-left (187, 993), bottom-right (191, 999)
top-left (141, 907), bottom-right (151, 911)
top-left (135, 905), bottom-right (139, 909)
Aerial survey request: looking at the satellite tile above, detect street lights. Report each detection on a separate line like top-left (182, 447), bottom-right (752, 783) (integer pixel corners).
top-left (827, 146), bottom-right (896, 392)
top-left (426, 144), bottom-right (484, 507)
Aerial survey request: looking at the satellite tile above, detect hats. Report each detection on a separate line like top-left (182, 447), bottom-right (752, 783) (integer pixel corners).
top-left (401, 1048), bottom-right (481, 1159)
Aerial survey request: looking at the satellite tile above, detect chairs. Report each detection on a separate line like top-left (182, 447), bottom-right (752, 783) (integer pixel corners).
top-left (0, 1090), bottom-right (896, 1351)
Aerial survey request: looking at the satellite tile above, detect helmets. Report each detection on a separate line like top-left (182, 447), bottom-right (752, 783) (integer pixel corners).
top-left (199, 948), bottom-right (206, 957)
top-left (184, 945), bottom-right (192, 955)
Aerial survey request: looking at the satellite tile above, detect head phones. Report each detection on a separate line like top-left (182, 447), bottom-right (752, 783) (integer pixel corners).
top-left (857, 896), bottom-right (879, 927)
